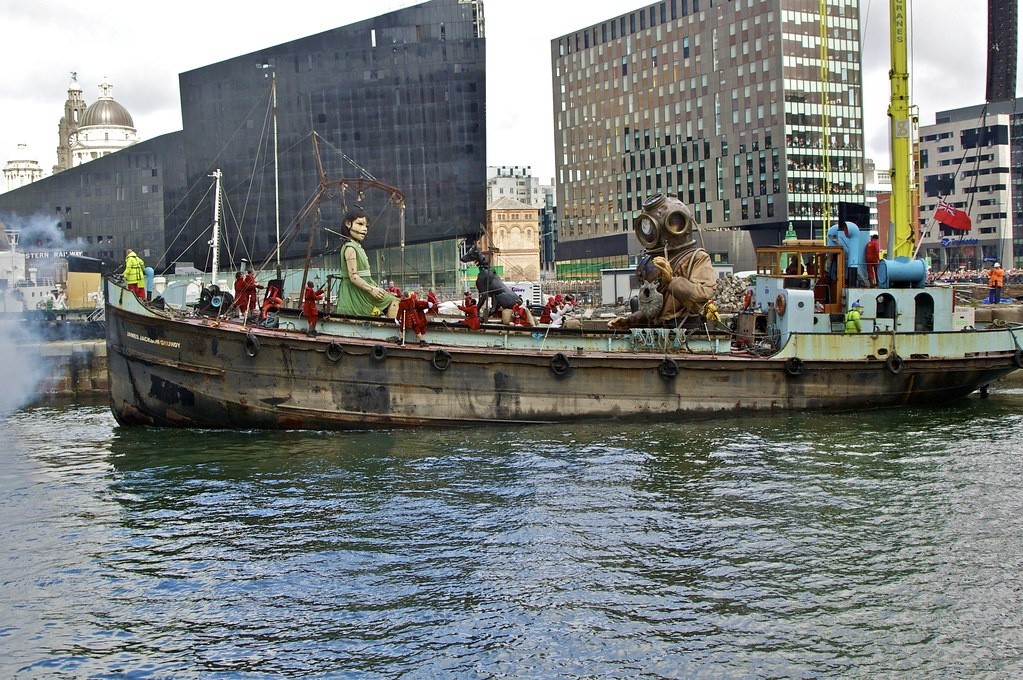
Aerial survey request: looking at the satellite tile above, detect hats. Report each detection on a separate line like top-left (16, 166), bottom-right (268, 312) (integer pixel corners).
top-left (871, 235), bottom-right (879, 239)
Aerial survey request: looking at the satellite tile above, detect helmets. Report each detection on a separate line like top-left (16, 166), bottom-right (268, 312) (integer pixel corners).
top-left (852, 302), bottom-right (860, 307)
top-left (994, 263), bottom-right (1000, 267)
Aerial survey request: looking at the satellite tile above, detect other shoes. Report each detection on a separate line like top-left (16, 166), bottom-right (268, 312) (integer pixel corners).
top-left (397, 338), bottom-right (403, 345)
top-left (419, 339), bottom-right (429, 347)
top-left (306, 330), bottom-right (320, 338)
top-left (443, 320), bottom-right (449, 328)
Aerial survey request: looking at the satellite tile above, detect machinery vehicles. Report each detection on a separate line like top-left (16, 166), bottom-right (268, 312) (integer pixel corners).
top-left (756, 0), bottom-right (924, 317)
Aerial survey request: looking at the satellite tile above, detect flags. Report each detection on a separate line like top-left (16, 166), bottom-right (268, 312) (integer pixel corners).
top-left (933, 200), bottom-right (972, 231)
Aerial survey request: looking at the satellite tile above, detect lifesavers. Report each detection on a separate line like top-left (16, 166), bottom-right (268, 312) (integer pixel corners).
top-left (244, 335), bottom-right (261, 358)
top-left (743, 290), bottom-right (752, 308)
top-left (325, 342), bottom-right (344, 363)
top-left (370, 344), bottom-right (388, 362)
top-left (431, 350), bottom-right (451, 371)
top-left (260, 303), bottom-right (281, 319)
top-left (775, 293), bottom-right (785, 316)
top-left (886, 354), bottom-right (904, 375)
top-left (785, 357), bottom-right (805, 376)
top-left (658, 359), bottom-right (679, 377)
top-left (550, 354), bottom-right (570, 376)
top-left (263, 297), bottom-right (283, 306)
top-left (1014, 349), bottom-right (1023, 369)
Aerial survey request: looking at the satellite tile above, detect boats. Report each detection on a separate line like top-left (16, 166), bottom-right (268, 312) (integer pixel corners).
top-left (102, 70), bottom-right (1022, 433)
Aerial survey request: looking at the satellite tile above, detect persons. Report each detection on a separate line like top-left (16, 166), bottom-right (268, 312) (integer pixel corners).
top-left (845, 302), bottom-right (864, 333)
top-left (386, 280), bottom-right (480, 347)
top-left (927, 267), bottom-right (1023, 284)
top-left (511, 298), bottom-right (531, 326)
top-left (303, 281), bottom-right (325, 338)
top-left (805, 257), bottom-right (821, 278)
top-left (539, 292), bottom-right (575, 327)
top-left (865, 234), bottom-right (880, 288)
top-left (822, 255), bottom-right (836, 304)
top-left (607, 192), bottom-right (716, 328)
top-left (988, 263), bottom-right (1006, 305)
top-left (336, 208), bottom-right (400, 319)
top-left (784, 255), bottom-right (805, 275)
top-left (233, 269), bottom-right (264, 320)
top-left (122, 249), bottom-right (146, 302)
